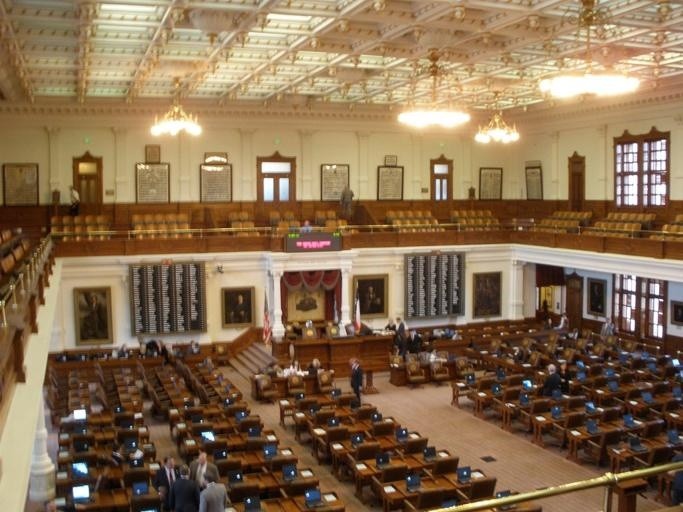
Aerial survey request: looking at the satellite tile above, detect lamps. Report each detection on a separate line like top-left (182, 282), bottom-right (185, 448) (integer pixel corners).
top-left (151, 92), bottom-right (201, 139)
top-left (397, 59), bottom-right (471, 131)
top-left (475, 94), bottom-right (521, 144)
top-left (540, 9), bottom-right (643, 100)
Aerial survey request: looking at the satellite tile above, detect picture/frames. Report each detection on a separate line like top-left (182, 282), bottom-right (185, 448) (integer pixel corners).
top-left (135, 163), bottom-right (171, 206)
top-left (670, 300), bottom-right (683, 325)
top-left (320, 163), bottom-right (350, 201)
top-left (586, 277), bottom-right (608, 317)
top-left (479, 167), bottom-right (503, 201)
top-left (204, 151), bottom-right (228, 166)
top-left (525, 166), bottom-right (543, 200)
top-left (376, 165), bottom-right (404, 200)
top-left (145, 145), bottom-right (160, 163)
top-left (471, 271), bottom-right (503, 317)
top-left (3, 162), bottom-right (40, 207)
top-left (384, 155), bottom-right (397, 167)
top-left (198, 165), bottom-right (232, 205)
top-left (219, 284), bottom-right (258, 328)
top-left (352, 272), bottom-right (390, 321)
top-left (71, 285), bottom-right (116, 344)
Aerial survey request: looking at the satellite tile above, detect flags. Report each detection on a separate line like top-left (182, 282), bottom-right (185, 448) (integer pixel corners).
top-left (263, 294), bottom-right (271, 344)
top-left (354, 298), bottom-right (361, 334)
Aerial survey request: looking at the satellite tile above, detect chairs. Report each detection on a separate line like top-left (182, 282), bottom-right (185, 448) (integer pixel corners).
top-left (1, 224), bottom-right (31, 287)
top-left (535, 210), bottom-right (594, 236)
top-left (451, 208), bottom-right (503, 231)
top-left (385, 210), bottom-right (445, 234)
top-left (647, 213), bottom-right (682, 242)
top-left (268, 208), bottom-right (302, 234)
top-left (580, 210), bottom-right (658, 239)
top-left (317, 208), bottom-right (350, 233)
top-left (228, 212), bottom-right (259, 237)
top-left (130, 212), bottom-right (190, 242)
top-left (47, 214), bottom-right (112, 242)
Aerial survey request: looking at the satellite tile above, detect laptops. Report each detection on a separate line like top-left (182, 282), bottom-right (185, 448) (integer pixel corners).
top-left (234, 411), bottom-right (245, 419)
top-left (264, 445), bottom-right (277, 458)
top-left (201, 431), bottom-right (214, 442)
top-left (333, 389), bottom-right (342, 396)
top-left (283, 464), bottom-right (296, 480)
top-left (248, 425), bottom-right (260, 436)
top-left (522, 379), bottom-right (533, 391)
top-left (72, 483), bottom-right (92, 501)
top-left (576, 371), bottom-right (585, 382)
top-left (73, 409), bottom-right (87, 421)
top-left (192, 414), bottom-right (205, 424)
top-left (228, 470), bottom-right (242, 482)
top-left (71, 462), bottom-right (91, 477)
top-left (679, 370), bottom-right (683, 378)
top-left (129, 460), bottom-right (146, 468)
top-left (648, 363), bottom-right (656, 373)
top-left (669, 428), bottom-right (681, 444)
top-left (397, 428), bottom-right (408, 440)
top-left (588, 421), bottom-right (600, 434)
top-left (672, 358), bottom-right (681, 367)
top-left (621, 354), bottom-right (628, 366)
top-left (376, 453), bottom-right (390, 467)
top-left (74, 426), bottom-right (88, 432)
top-left (640, 353), bottom-right (648, 361)
top-left (124, 439), bottom-right (137, 451)
top-left (640, 392), bottom-right (653, 402)
top-left (350, 399), bottom-right (359, 407)
top-left (608, 381), bottom-right (618, 390)
top-left (604, 368), bottom-right (612, 377)
top-left (304, 488), bottom-right (324, 507)
top-left (456, 467), bottom-right (471, 483)
top-left (467, 373), bottom-right (475, 384)
top-left (73, 438), bottom-right (87, 452)
top-left (225, 398), bottom-right (233, 406)
top-left (672, 385), bottom-right (683, 399)
top-left (407, 472), bottom-right (423, 492)
top-left (585, 401), bottom-right (596, 413)
top-left (497, 372), bottom-right (506, 380)
top-left (603, 351), bottom-right (609, 360)
top-left (518, 395), bottom-right (530, 405)
top-left (423, 446), bottom-right (437, 461)
top-left (185, 402), bottom-right (191, 406)
top-left (552, 405), bottom-right (562, 418)
top-left (131, 482), bottom-right (147, 495)
top-left (630, 436), bottom-right (647, 452)
top-left (121, 421), bottom-right (133, 428)
top-left (576, 361), bottom-right (585, 370)
top-left (372, 413), bottom-right (383, 423)
top-left (114, 405), bottom-right (123, 413)
top-left (493, 384), bottom-right (504, 394)
top-left (214, 447), bottom-right (227, 459)
top-left (328, 417), bottom-right (338, 426)
top-left (623, 413), bottom-right (637, 428)
top-left (353, 434), bottom-right (363, 444)
top-left (297, 392), bottom-right (304, 400)
top-left (310, 402), bottom-right (319, 409)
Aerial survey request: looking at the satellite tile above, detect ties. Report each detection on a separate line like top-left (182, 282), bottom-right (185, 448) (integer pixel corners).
top-left (170, 469), bottom-right (174, 486)
top-left (197, 464), bottom-right (203, 484)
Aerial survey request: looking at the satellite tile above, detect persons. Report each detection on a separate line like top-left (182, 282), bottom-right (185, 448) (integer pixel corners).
top-left (539, 364), bottom-right (562, 395)
top-left (542, 300), bottom-right (548, 314)
top-left (56, 341), bottom-right (198, 360)
top-left (294, 322), bottom-right (301, 336)
top-left (80, 293), bottom-right (107, 341)
top-left (340, 184), bottom-right (352, 218)
top-left (559, 363), bottom-right (573, 394)
top-left (326, 321), bottom-right (340, 338)
top-left (370, 297), bottom-right (382, 312)
top-left (348, 358), bottom-right (364, 408)
top-left (364, 285), bottom-right (375, 313)
top-left (156, 452), bottom-right (226, 512)
top-left (554, 313), bottom-right (569, 330)
top-left (307, 358), bottom-right (321, 373)
top-left (69, 185), bottom-right (80, 213)
top-left (385, 317), bottom-right (424, 355)
top-left (600, 317), bottom-right (614, 336)
top-left (300, 220), bottom-right (312, 234)
